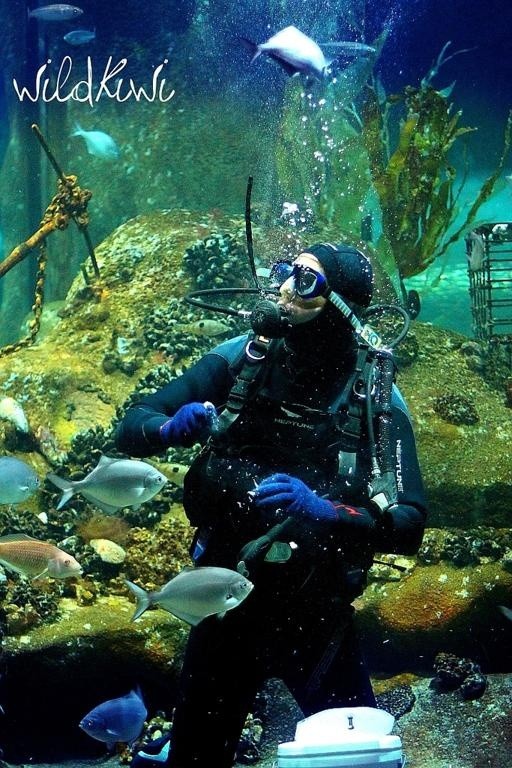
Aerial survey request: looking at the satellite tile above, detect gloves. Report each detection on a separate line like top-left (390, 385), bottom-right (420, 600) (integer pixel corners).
top-left (160, 401), bottom-right (210, 444)
top-left (254, 473), bottom-right (337, 525)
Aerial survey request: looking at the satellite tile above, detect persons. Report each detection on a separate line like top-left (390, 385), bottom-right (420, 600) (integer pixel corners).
top-left (114, 241), bottom-right (430, 768)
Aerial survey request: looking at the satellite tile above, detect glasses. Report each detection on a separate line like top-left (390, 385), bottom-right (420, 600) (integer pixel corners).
top-left (268, 259), bottom-right (364, 321)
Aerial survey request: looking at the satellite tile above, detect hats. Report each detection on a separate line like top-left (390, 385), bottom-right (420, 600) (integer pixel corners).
top-left (283, 242), bottom-right (374, 356)
